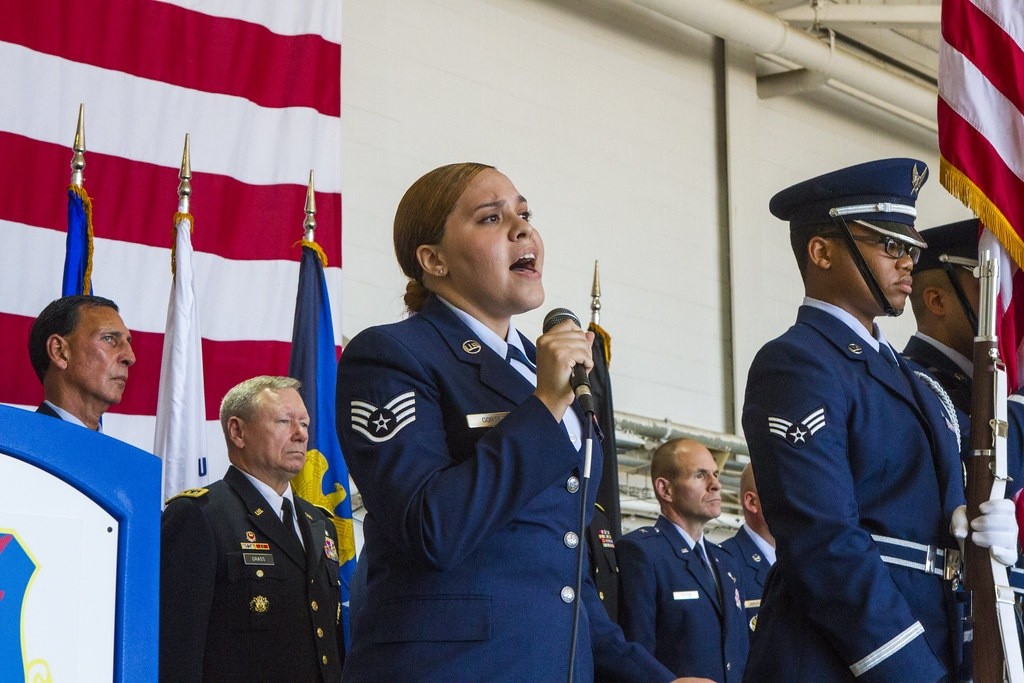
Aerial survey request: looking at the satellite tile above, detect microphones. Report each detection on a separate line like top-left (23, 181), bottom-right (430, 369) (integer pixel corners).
top-left (543, 308), bottom-right (595, 417)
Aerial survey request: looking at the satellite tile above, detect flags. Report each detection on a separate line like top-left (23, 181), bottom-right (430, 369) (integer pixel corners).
top-left (941, 3), bottom-right (1023, 609)
top-left (0, 0), bottom-right (622, 683)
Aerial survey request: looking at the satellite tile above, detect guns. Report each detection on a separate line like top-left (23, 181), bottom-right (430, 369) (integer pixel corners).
top-left (964, 249), bottom-right (1023, 683)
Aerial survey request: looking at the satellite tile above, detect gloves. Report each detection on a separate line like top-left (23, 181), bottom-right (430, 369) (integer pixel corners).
top-left (951, 499), bottom-right (1019, 566)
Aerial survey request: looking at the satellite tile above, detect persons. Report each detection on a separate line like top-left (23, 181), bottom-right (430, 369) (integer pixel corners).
top-left (335, 166), bottom-right (716, 683)
top-left (904, 218), bottom-right (1024, 682)
top-left (742, 164), bottom-right (1018, 683)
top-left (28, 294), bottom-right (777, 683)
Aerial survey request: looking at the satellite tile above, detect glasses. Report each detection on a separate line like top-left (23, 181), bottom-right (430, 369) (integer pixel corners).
top-left (821, 232), bottom-right (921, 264)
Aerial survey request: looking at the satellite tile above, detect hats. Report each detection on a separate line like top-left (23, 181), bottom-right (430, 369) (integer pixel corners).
top-left (911, 218), bottom-right (980, 275)
top-left (769, 158), bottom-right (929, 249)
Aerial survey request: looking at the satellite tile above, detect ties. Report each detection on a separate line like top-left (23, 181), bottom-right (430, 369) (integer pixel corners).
top-left (281, 497), bottom-right (305, 555)
top-left (694, 543), bottom-right (713, 577)
top-left (879, 343), bottom-right (912, 394)
